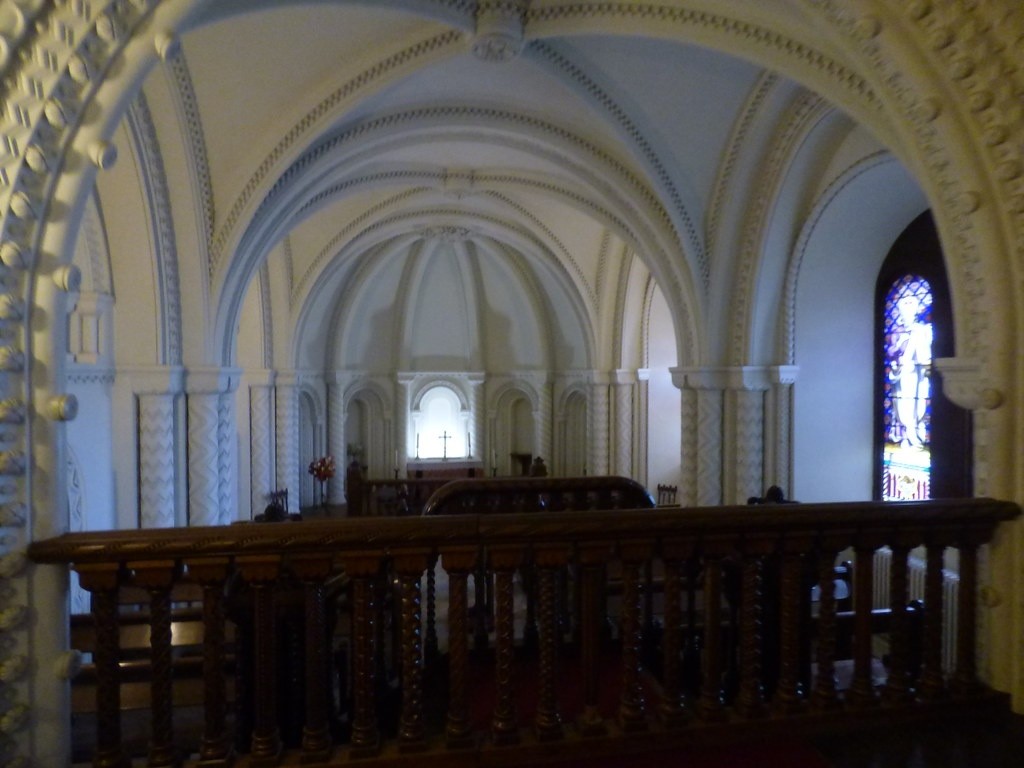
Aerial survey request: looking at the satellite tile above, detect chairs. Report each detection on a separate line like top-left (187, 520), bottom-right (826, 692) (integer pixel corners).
top-left (807, 564), bottom-right (853, 611)
top-left (808, 599), bottom-right (927, 694)
